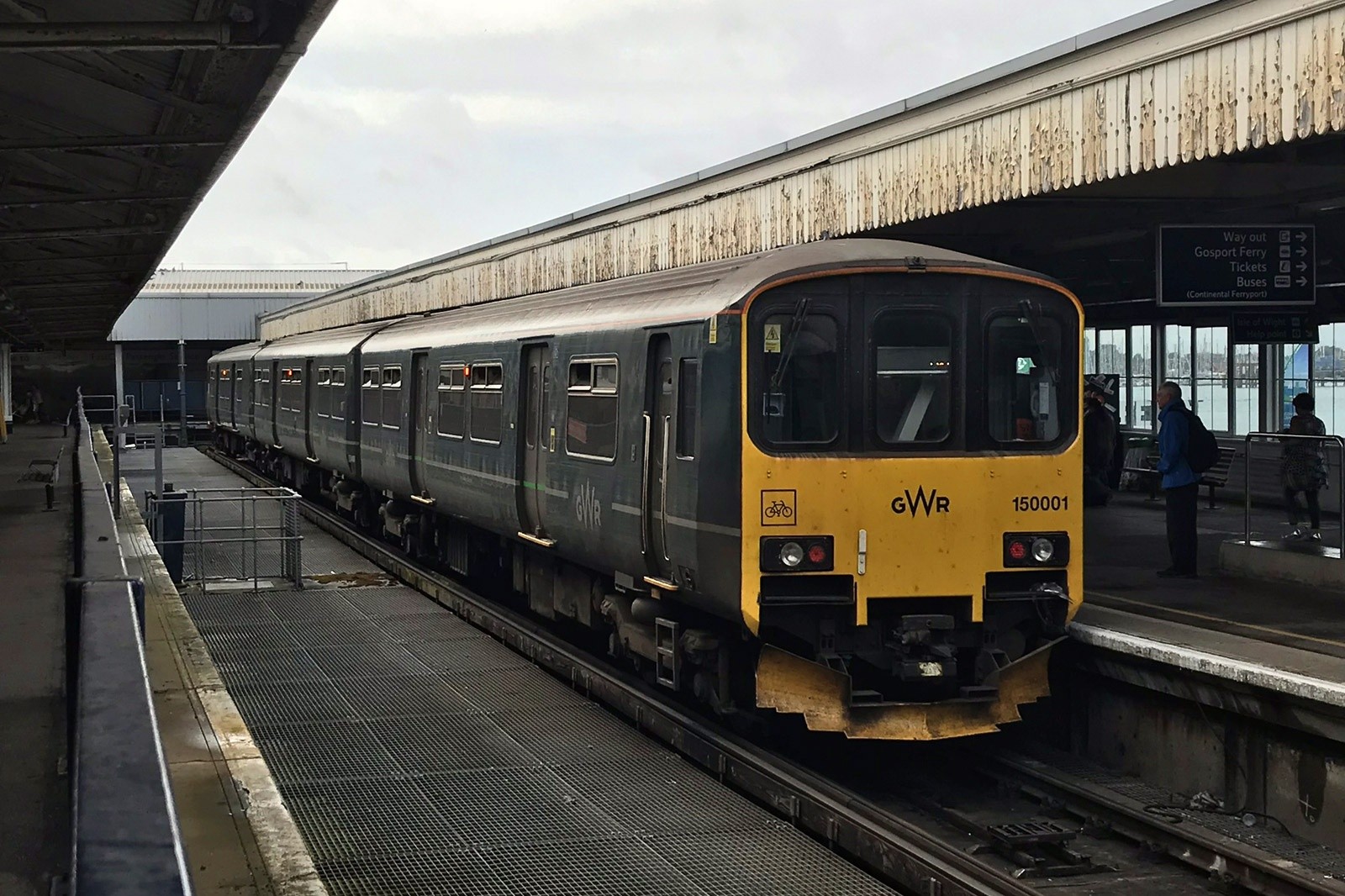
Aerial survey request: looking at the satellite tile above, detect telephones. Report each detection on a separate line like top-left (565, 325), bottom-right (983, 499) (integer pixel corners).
top-left (1030, 390), bottom-right (1039, 415)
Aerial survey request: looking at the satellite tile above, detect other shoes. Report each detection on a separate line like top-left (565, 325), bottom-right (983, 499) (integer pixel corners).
top-left (1156, 564), bottom-right (1194, 578)
top-left (1282, 528), bottom-right (1301, 540)
top-left (1302, 532), bottom-right (1322, 541)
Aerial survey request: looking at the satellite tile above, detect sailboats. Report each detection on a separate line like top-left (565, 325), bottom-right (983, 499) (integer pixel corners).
top-left (1117, 336), bottom-right (1226, 388)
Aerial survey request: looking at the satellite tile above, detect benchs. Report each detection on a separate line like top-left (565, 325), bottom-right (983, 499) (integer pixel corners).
top-left (17, 444), bottom-right (65, 512)
top-left (59, 408), bottom-right (72, 437)
top-left (1124, 441), bottom-right (1238, 509)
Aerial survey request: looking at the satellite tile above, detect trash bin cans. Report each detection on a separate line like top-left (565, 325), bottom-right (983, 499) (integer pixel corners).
top-left (150, 489), bottom-right (188, 587)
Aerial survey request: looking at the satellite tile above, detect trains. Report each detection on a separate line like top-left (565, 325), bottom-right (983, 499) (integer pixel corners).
top-left (205, 238), bottom-right (1086, 800)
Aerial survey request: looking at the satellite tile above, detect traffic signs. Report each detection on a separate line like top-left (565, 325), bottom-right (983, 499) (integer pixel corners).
top-left (1159, 225), bottom-right (1317, 307)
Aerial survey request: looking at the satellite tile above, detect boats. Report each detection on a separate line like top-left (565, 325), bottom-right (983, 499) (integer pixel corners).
top-left (1139, 398), bottom-right (1205, 422)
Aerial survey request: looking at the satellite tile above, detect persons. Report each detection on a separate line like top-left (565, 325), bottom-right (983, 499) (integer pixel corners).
top-left (1276, 393), bottom-right (1327, 543)
top-left (761, 312), bottom-right (841, 443)
top-left (1083, 390), bottom-right (1120, 507)
top-left (25, 386), bottom-right (44, 424)
top-left (1155, 381), bottom-right (1202, 578)
top-left (983, 314), bottom-right (1072, 442)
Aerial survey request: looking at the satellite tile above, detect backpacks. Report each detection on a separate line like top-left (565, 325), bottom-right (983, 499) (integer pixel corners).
top-left (1167, 406), bottom-right (1220, 472)
